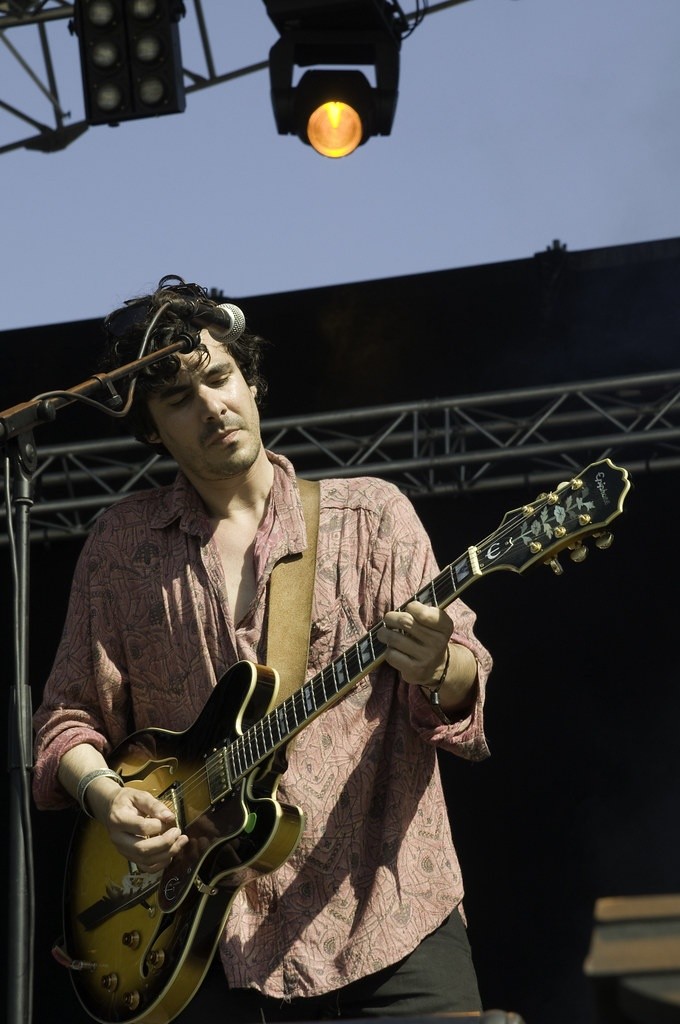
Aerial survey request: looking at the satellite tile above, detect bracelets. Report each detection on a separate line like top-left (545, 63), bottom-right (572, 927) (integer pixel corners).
top-left (418, 644), bottom-right (450, 706)
top-left (77, 769), bottom-right (125, 821)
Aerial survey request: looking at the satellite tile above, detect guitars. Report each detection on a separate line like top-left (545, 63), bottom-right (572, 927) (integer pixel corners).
top-left (58, 457), bottom-right (632, 1024)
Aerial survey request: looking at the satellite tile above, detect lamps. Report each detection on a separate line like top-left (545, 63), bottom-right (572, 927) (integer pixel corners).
top-left (260, 0), bottom-right (410, 160)
top-left (67, 0), bottom-right (186, 128)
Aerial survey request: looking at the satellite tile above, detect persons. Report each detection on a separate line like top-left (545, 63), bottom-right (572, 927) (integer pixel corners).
top-left (35, 274), bottom-right (494, 1024)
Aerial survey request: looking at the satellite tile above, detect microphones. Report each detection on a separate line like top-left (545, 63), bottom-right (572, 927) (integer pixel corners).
top-left (168, 297), bottom-right (246, 344)
